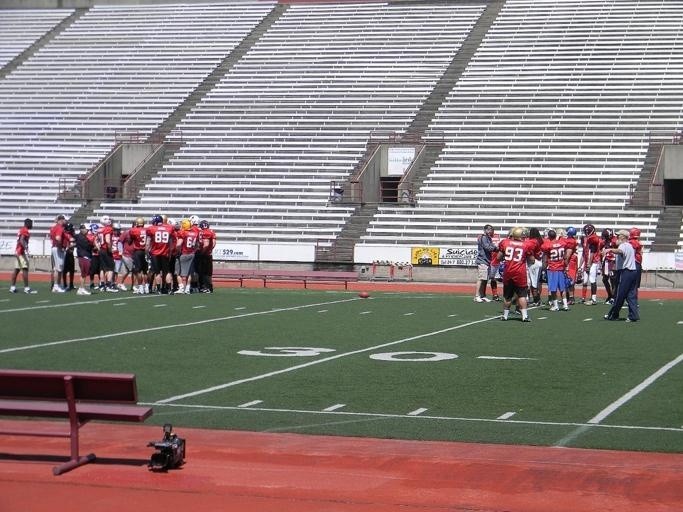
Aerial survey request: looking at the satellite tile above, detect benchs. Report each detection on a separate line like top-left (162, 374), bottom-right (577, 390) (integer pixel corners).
top-left (211, 270), bottom-right (362, 291)
top-left (1, 367), bottom-right (154, 475)
top-left (2, 3), bottom-right (682, 250)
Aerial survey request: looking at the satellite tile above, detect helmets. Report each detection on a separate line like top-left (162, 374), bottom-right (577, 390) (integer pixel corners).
top-left (483, 224), bottom-right (642, 240)
top-left (90, 215), bottom-right (210, 233)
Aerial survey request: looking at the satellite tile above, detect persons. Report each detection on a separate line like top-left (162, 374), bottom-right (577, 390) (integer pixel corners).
top-left (473, 224), bottom-right (642, 321)
top-left (9, 219), bottom-right (38, 294)
top-left (49, 214), bottom-right (217, 296)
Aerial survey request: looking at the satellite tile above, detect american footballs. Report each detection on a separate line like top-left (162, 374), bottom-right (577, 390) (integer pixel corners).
top-left (358, 292), bottom-right (370, 298)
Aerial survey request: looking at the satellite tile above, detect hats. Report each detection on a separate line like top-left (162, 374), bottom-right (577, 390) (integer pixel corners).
top-left (57, 215), bottom-right (86, 230)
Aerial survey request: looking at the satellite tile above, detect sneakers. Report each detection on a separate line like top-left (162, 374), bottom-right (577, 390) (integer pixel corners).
top-left (9, 285), bottom-right (213, 295)
top-left (472, 294), bottom-right (640, 322)
